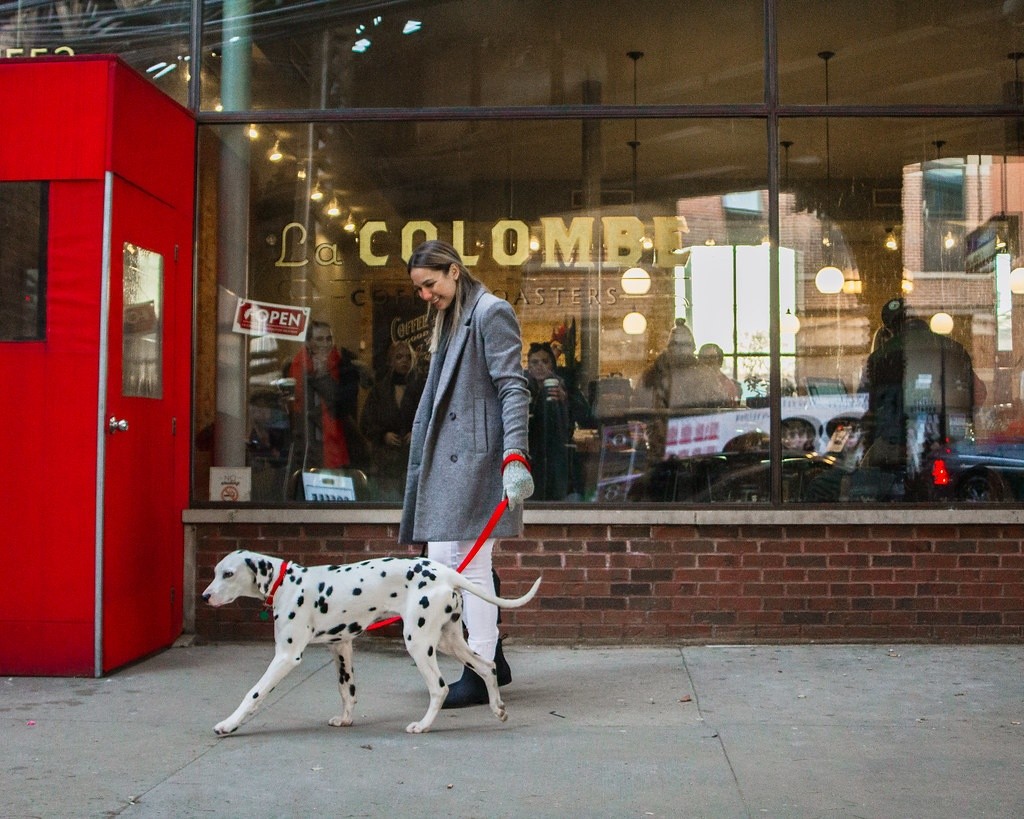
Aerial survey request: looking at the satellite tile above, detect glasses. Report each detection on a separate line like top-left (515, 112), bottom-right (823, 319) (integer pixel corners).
top-left (698, 355), bottom-right (722, 360)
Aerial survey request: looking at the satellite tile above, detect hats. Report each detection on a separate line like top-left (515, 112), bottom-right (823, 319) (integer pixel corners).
top-left (665, 317), bottom-right (697, 356)
top-left (881, 297), bottom-right (912, 328)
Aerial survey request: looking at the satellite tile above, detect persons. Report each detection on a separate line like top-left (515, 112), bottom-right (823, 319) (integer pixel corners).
top-left (861, 295), bottom-right (987, 445)
top-left (398, 242), bottom-right (534, 710)
top-left (283, 318), bottom-right (738, 503)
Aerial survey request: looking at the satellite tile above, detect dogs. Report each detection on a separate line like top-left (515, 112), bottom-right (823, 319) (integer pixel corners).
top-left (202, 550), bottom-right (541, 737)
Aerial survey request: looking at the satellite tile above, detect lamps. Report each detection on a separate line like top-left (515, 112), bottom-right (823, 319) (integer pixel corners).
top-left (621, 51), bottom-right (651, 295)
top-left (1006, 51), bottom-right (1024, 295)
top-left (929, 139), bottom-right (954, 336)
top-left (780, 141), bottom-right (801, 334)
top-left (621, 142), bottom-right (648, 335)
top-left (814, 50), bottom-right (845, 294)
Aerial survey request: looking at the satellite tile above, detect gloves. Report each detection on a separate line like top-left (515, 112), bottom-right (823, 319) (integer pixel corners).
top-left (501, 448), bottom-right (535, 512)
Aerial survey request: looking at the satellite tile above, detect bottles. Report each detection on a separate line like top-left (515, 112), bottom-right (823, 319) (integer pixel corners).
top-left (840, 475), bottom-right (848, 501)
top-left (740, 484), bottom-right (758, 502)
top-left (783, 471), bottom-right (811, 503)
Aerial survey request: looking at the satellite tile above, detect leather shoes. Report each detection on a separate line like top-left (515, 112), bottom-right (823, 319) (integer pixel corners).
top-left (441, 664), bottom-right (490, 708)
top-left (493, 633), bottom-right (513, 687)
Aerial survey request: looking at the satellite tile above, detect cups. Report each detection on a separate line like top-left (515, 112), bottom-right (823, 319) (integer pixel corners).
top-left (544, 378), bottom-right (559, 401)
top-left (891, 479), bottom-right (904, 503)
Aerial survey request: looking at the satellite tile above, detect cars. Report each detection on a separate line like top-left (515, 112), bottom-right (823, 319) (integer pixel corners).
top-left (920, 437), bottom-right (1024, 502)
top-left (637, 373), bottom-right (872, 472)
